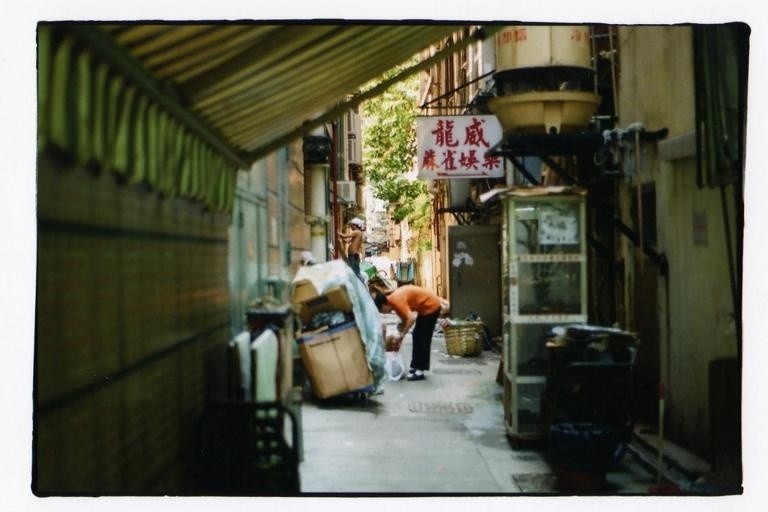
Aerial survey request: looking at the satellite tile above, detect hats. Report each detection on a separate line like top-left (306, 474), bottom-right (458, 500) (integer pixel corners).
top-left (348, 218), bottom-right (365, 230)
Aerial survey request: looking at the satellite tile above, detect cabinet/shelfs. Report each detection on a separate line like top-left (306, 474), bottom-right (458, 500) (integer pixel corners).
top-left (499, 185), bottom-right (589, 450)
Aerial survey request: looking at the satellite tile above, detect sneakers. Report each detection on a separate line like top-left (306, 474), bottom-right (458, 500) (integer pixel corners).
top-left (406, 369), bottom-right (425, 380)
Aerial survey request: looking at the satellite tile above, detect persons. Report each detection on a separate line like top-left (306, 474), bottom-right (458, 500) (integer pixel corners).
top-left (337, 217), bottom-right (366, 284)
top-left (373, 285), bottom-right (442, 381)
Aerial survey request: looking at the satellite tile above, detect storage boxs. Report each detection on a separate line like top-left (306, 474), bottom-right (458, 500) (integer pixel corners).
top-left (289, 260), bottom-right (382, 401)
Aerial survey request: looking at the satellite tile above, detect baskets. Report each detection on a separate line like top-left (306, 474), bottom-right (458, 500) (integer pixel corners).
top-left (444, 321), bottom-right (486, 356)
